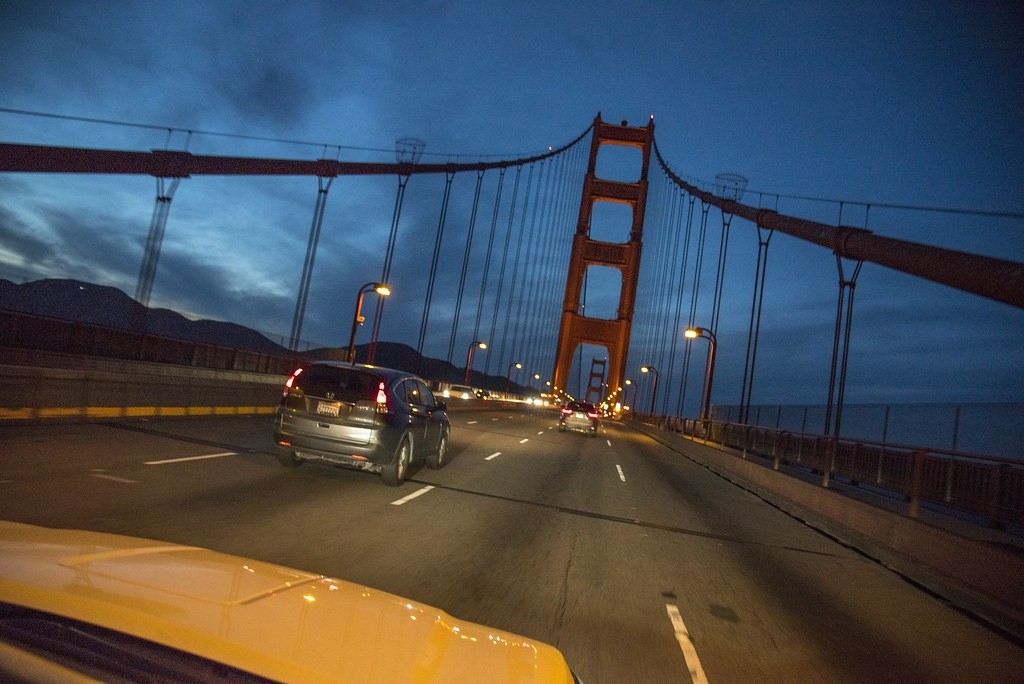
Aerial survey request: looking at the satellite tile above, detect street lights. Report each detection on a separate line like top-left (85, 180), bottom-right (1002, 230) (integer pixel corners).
top-left (597, 378), bottom-right (639, 419)
top-left (525, 373), bottom-right (575, 407)
top-left (640, 366), bottom-right (659, 419)
top-left (683, 327), bottom-right (717, 427)
top-left (347, 282), bottom-right (391, 364)
top-left (504, 362), bottom-right (522, 400)
top-left (464, 341), bottom-right (487, 384)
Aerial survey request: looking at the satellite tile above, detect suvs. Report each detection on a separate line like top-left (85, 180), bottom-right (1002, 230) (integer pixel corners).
top-left (273, 363), bottom-right (454, 485)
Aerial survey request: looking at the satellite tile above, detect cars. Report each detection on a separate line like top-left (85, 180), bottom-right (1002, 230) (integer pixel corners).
top-left (444, 385), bottom-right (478, 401)
top-left (558, 400), bottom-right (598, 436)
